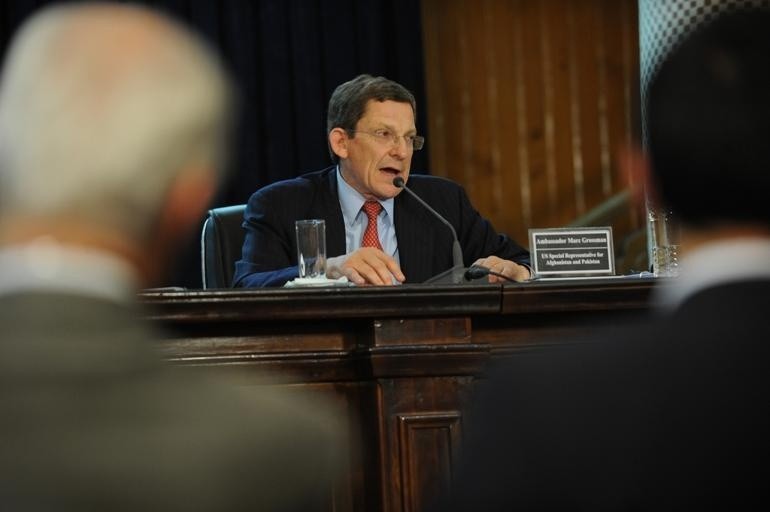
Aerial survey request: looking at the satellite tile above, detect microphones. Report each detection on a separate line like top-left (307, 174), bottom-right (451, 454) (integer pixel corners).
top-left (393, 175), bottom-right (489, 285)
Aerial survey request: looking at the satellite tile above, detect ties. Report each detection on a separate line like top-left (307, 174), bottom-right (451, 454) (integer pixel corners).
top-left (360, 199), bottom-right (385, 285)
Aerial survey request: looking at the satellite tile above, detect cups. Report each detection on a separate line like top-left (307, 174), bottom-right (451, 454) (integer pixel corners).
top-left (295, 219), bottom-right (327, 279)
top-left (642, 194), bottom-right (679, 279)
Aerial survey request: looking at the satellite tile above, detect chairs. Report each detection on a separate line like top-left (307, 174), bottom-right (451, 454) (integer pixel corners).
top-left (197, 202), bottom-right (251, 293)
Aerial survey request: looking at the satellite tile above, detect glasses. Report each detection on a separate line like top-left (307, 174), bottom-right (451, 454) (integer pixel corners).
top-left (345, 129), bottom-right (427, 151)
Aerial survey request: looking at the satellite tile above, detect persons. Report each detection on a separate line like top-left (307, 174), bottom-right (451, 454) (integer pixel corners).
top-left (228, 73), bottom-right (535, 289)
top-left (415, 1), bottom-right (769, 511)
top-left (0, 1), bottom-right (367, 511)
